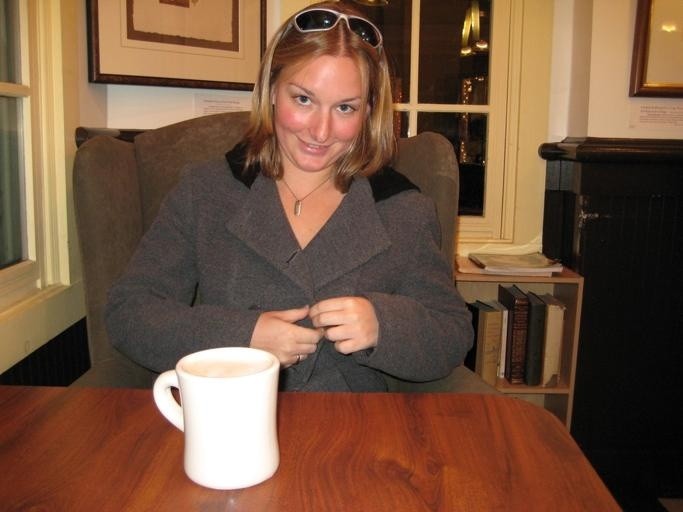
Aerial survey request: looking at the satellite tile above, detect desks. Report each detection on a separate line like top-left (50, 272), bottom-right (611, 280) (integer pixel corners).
top-left (0, 385), bottom-right (623, 512)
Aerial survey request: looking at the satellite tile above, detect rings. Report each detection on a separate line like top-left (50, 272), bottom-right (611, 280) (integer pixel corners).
top-left (293, 355), bottom-right (301, 366)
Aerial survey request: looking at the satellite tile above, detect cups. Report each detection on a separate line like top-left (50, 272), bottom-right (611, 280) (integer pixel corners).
top-left (151, 345), bottom-right (282, 493)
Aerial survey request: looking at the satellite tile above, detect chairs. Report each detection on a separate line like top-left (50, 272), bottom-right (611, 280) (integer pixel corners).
top-left (67, 109), bottom-right (504, 393)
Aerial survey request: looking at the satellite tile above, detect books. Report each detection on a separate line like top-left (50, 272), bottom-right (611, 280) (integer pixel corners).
top-left (465, 283), bottom-right (568, 388)
top-left (456, 255), bottom-right (553, 278)
top-left (468, 250), bottom-right (563, 272)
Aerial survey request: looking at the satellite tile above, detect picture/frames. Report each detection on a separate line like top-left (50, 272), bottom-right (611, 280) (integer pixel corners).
top-left (629, 0), bottom-right (683, 98)
top-left (86, 0), bottom-right (267, 91)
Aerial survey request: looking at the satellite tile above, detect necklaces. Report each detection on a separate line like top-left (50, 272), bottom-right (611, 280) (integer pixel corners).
top-left (282, 174), bottom-right (332, 217)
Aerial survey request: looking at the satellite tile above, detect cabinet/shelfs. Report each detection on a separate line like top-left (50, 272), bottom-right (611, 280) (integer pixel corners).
top-left (541, 138), bottom-right (683, 498)
top-left (455, 256), bottom-right (585, 434)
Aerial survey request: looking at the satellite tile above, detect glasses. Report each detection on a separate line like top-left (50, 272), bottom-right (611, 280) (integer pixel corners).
top-left (280, 8), bottom-right (383, 56)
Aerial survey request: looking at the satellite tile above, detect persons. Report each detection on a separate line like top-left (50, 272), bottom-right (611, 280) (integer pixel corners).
top-left (101, 0), bottom-right (479, 393)
